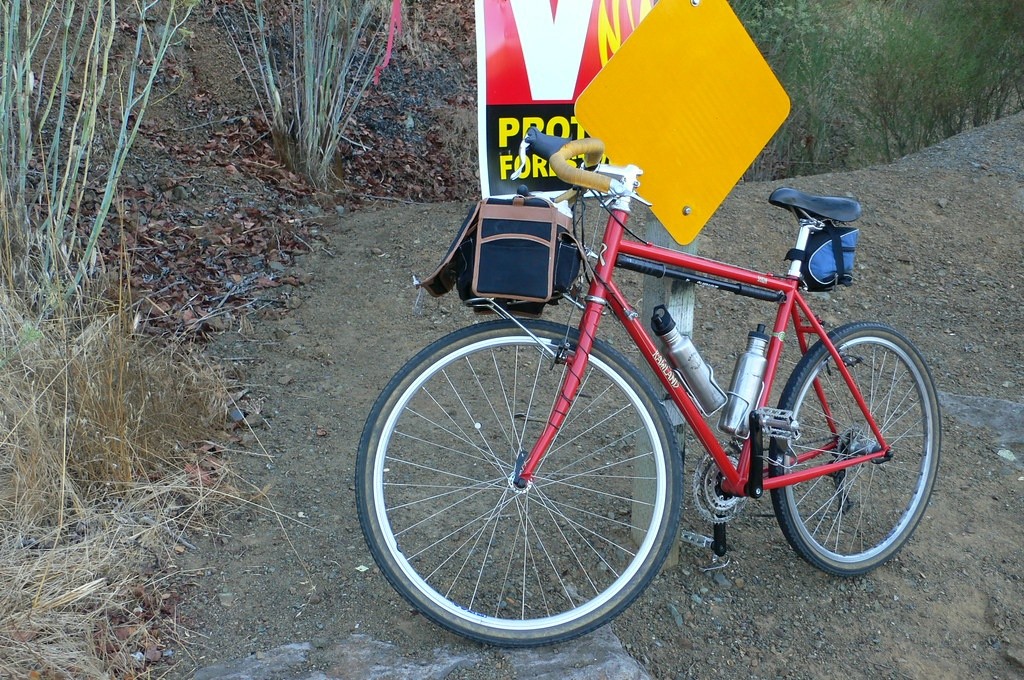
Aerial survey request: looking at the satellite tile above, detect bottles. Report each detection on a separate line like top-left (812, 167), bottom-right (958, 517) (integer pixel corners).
top-left (718, 324), bottom-right (770, 438)
top-left (650, 304), bottom-right (727, 414)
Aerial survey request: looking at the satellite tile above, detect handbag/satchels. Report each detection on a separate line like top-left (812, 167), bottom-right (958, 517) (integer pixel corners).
top-left (415, 195), bottom-right (599, 319)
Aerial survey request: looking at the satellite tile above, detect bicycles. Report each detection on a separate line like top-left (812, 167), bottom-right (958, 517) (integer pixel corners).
top-left (354, 136), bottom-right (940, 648)
